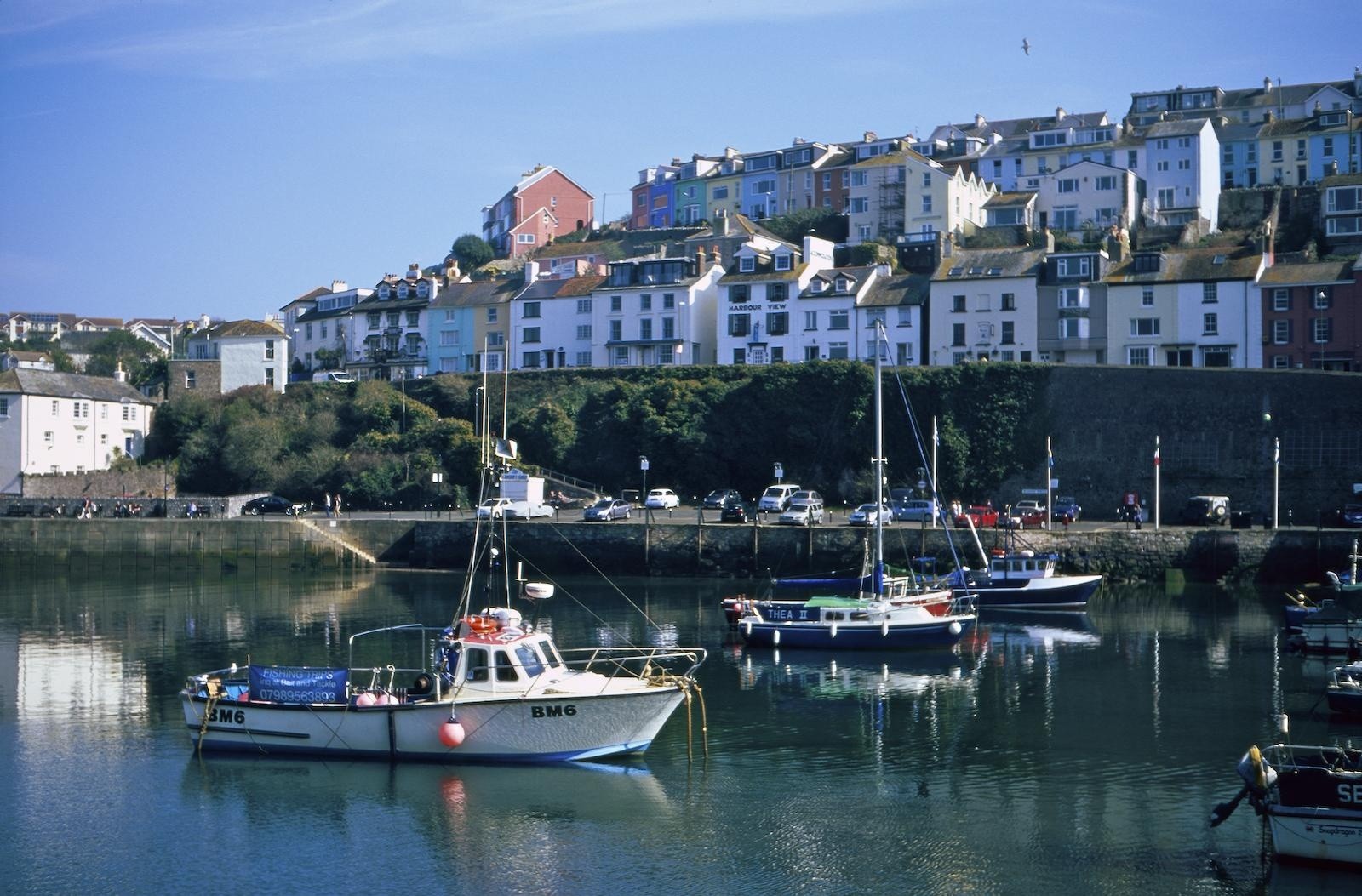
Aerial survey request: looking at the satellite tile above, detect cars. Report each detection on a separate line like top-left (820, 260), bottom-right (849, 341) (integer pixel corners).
top-left (848, 503), bottom-right (893, 526)
top-left (476, 487), bottom-right (760, 524)
top-left (1052, 503), bottom-right (1079, 522)
top-left (1326, 503), bottom-right (1362, 527)
top-left (789, 490), bottom-right (823, 505)
top-left (244, 495), bottom-right (308, 516)
top-left (779, 502), bottom-right (824, 526)
top-left (892, 500), bottom-right (946, 522)
top-left (999, 508), bottom-right (1049, 529)
top-left (953, 507), bottom-right (999, 529)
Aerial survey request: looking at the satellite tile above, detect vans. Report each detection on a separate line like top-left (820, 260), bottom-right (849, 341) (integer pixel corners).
top-left (758, 484), bottom-right (802, 513)
top-left (311, 370), bottom-right (354, 383)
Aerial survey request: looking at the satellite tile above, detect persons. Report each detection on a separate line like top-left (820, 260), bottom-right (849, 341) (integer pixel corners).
top-left (1135, 504), bottom-right (1142, 530)
top-left (77, 495), bottom-right (201, 520)
top-left (950, 501), bottom-right (962, 521)
top-left (435, 626), bottom-right (459, 688)
top-left (325, 493), bottom-right (343, 517)
top-left (550, 490), bottom-right (613, 508)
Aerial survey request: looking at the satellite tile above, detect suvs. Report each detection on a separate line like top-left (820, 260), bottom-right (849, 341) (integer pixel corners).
top-left (1179, 496), bottom-right (1231, 526)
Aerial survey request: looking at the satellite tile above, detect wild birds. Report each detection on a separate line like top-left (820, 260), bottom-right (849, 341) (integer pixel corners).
top-left (1022, 36), bottom-right (1031, 56)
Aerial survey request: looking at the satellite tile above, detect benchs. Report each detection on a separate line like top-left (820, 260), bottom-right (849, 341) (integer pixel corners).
top-left (185, 505), bottom-right (212, 517)
top-left (6, 505), bottom-right (34, 518)
top-left (114, 507), bottom-right (142, 518)
top-left (75, 504), bottom-right (106, 518)
top-left (40, 505), bottom-right (67, 517)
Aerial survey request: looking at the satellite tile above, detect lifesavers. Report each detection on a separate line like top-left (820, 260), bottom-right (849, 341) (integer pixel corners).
top-left (991, 555), bottom-right (1005, 559)
top-left (991, 549), bottom-right (1005, 554)
top-left (459, 617), bottom-right (497, 629)
top-left (470, 627), bottom-right (498, 634)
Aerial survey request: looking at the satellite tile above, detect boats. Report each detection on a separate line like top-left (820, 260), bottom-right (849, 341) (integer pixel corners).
top-left (1237, 743), bottom-right (1362, 864)
top-left (177, 333), bottom-right (709, 770)
top-left (1285, 571), bottom-right (1362, 655)
top-left (1324, 660), bottom-right (1362, 712)
top-left (906, 505), bottom-right (1102, 613)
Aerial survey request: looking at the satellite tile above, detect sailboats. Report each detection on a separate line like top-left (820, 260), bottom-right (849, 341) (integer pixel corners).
top-left (719, 319), bottom-right (980, 653)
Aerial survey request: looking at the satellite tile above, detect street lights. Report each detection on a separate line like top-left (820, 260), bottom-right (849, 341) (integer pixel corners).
top-left (1263, 413), bottom-right (1280, 528)
top-left (432, 464), bottom-right (442, 518)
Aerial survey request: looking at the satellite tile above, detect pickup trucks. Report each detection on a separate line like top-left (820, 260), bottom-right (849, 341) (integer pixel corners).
top-left (1016, 500), bottom-right (1046, 511)
